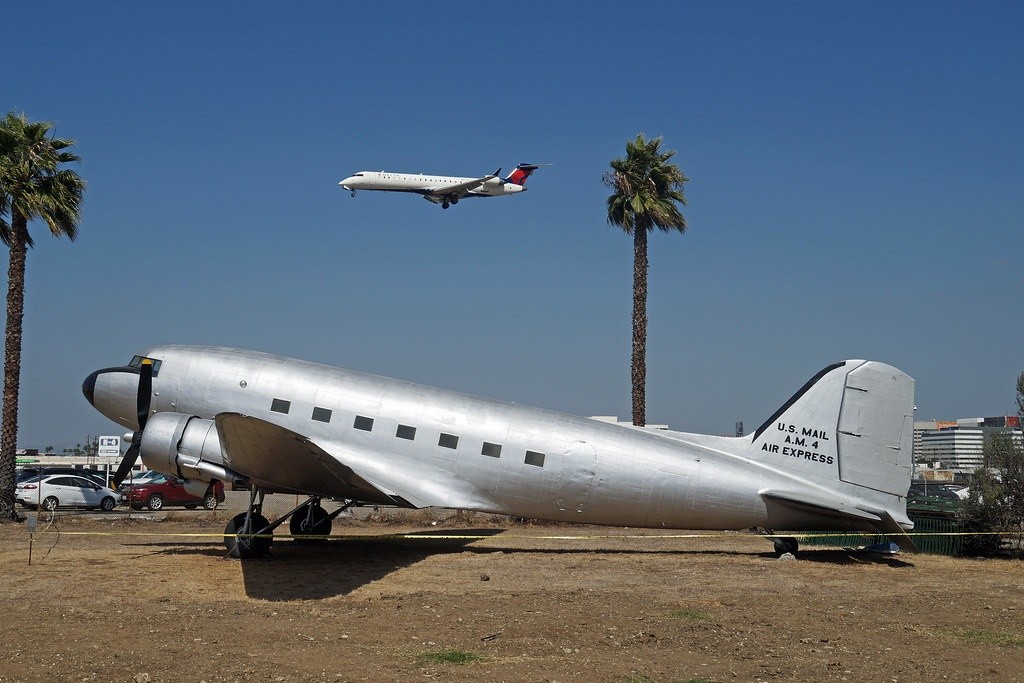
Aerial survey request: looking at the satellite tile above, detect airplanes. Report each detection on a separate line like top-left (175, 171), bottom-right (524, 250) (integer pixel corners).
top-left (337, 159), bottom-right (557, 213)
top-left (81, 341), bottom-right (922, 560)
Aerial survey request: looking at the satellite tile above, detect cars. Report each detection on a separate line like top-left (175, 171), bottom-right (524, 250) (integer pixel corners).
top-left (15, 467), bottom-right (161, 503)
top-left (125, 474), bottom-right (227, 512)
top-left (13, 473), bottom-right (122, 512)
